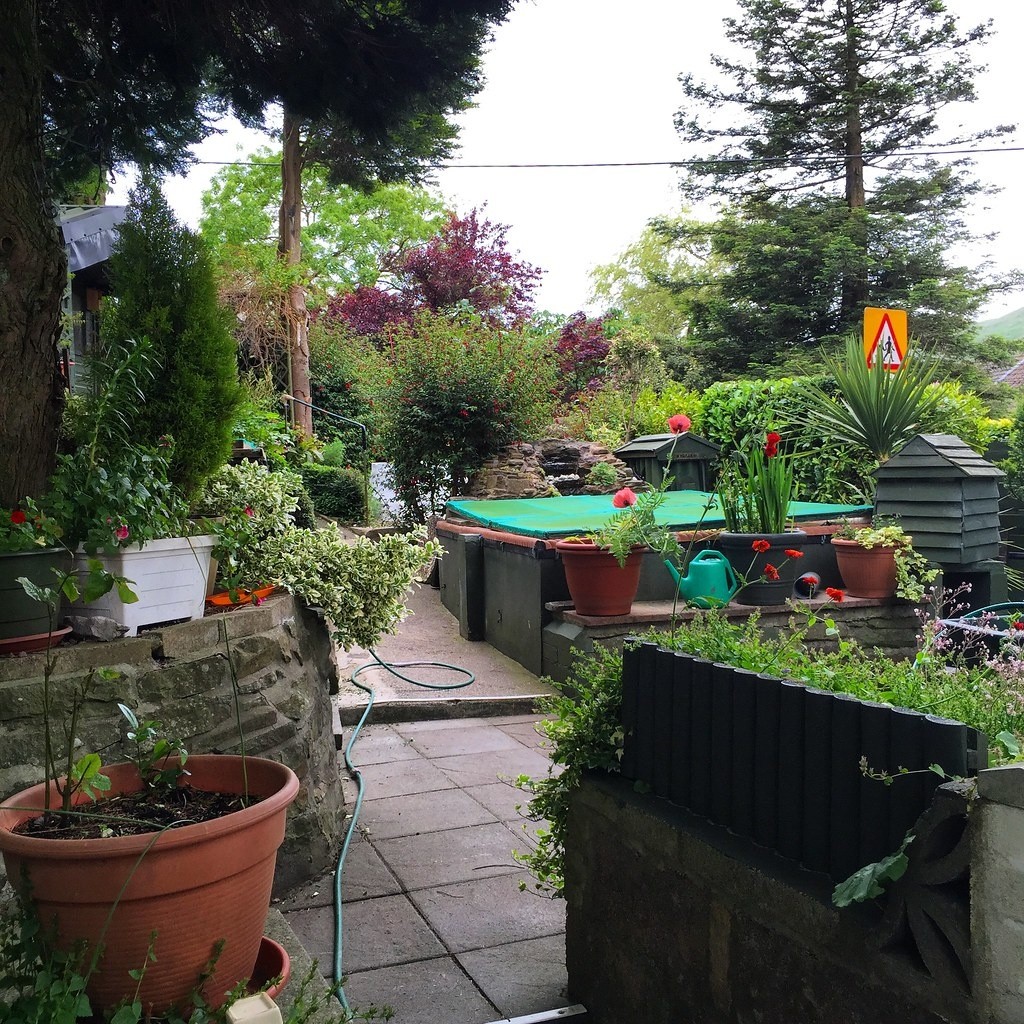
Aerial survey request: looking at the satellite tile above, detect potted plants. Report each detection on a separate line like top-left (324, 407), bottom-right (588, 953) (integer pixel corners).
top-left (718, 433), bottom-right (806, 606)
top-left (0, 497), bottom-right (297, 1023)
top-left (832, 520), bottom-right (942, 603)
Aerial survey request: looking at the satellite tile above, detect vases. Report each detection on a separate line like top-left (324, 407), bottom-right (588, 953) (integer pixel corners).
top-left (70, 532), bottom-right (219, 641)
top-left (556, 540), bottom-right (648, 616)
top-left (1, 550), bottom-right (78, 653)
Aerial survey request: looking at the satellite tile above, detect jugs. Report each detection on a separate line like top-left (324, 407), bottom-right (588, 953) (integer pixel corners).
top-left (664, 550), bottom-right (737, 608)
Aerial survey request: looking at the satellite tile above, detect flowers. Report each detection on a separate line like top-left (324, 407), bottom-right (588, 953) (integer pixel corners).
top-left (576, 413), bottom-right (701, 561)
top-left (0, 348), bottom-right (208, 551)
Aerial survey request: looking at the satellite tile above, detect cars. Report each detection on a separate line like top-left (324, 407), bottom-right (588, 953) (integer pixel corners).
top-left (933, 602), bottom-right (1024, 677)
